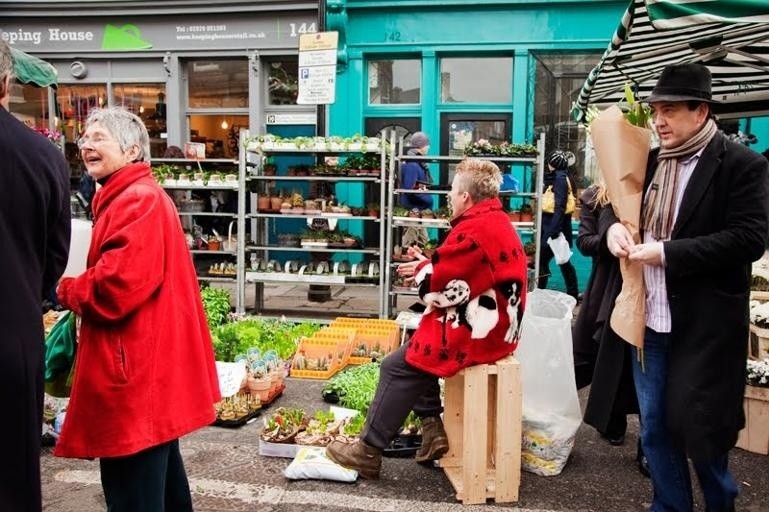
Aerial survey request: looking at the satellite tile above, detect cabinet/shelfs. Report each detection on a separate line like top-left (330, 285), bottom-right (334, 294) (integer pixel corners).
top-left (150, 157), bottom-right (240, 314)
top-left (386, 129), bottom-right (546, 319)
top-left (239, 126), bottom-right (386, 324)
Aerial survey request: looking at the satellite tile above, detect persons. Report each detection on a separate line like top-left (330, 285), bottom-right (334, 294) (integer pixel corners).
top-left (572, 147), bottom-right (662, 477)
top-left (400, 132), bottom-right (434, 210)
top-left (209, 141), bottom-right (224, 157)
top-left (600, 63), bottom-right (769, 512)
top-left (229, 141), bottom-right (238, 158)
top-left (1, 42), bottom-right (71, 511)
top-left (539, 149), bottom-right (579, 309)
top-left (54, 107), bottom-right (221, 512)
top-left (324, 160), bottom-right (528, 480)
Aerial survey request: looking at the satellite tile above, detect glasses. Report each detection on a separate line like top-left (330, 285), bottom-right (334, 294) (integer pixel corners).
top-left (77, 137), bottom-right (118, 150)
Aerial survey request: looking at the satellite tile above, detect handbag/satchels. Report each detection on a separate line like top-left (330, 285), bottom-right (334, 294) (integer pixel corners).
top-left (542, 185), bottom-right (576, 214)
top-left (44, 310), bottom-right (76, 397)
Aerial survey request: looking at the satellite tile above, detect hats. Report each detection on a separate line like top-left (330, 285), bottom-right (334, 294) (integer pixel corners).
top-left (549, 151), bottom-right (568, 170)
top-left (638, 63), bottom-right (720, 104)
top-left (411, 132), bottom-right (427, 148)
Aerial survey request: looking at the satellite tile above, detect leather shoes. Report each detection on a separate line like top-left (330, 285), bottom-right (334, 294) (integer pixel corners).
top-left (610, 435), bottom-right (652, 478)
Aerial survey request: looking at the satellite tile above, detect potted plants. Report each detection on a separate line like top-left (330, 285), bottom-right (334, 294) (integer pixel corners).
top-left (261, 407), bottom-right (360, 446)
top-left (234, 347), bottom-right (287, 401)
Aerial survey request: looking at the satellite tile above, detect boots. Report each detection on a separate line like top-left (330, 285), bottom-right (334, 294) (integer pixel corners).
top-left (326, 441), bottom-right (382, 479)
top-left (415, 417), bottom-right (449, 462)
